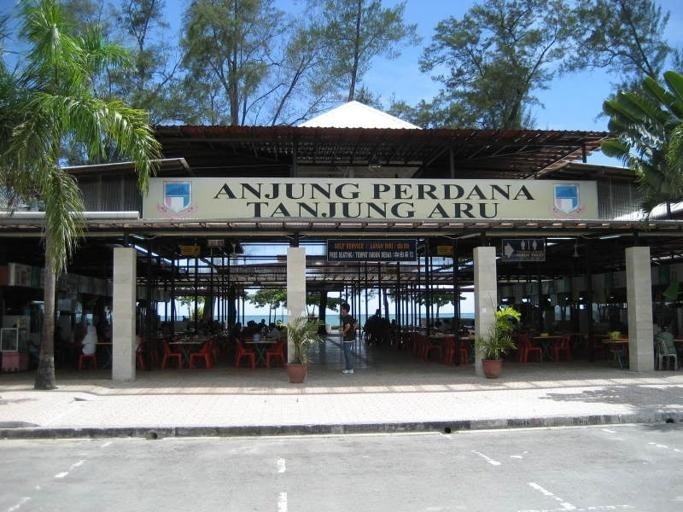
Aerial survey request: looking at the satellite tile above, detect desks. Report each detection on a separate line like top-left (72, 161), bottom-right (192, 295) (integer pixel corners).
top-left (601, 339), bottom-right (628, 368)
top-left (93, 340), bottom-right (112, 369)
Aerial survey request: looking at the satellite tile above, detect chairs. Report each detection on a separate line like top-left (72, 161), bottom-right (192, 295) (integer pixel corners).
top-left (413, 327), bottom-right (573, 368)
top-left (653, 337), bottom-right (680, 372)
top-left (136, 335), bottom-right (286, 369)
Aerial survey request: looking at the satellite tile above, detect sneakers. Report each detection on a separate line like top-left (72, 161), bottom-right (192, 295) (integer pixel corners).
top-left (342, 368), bottom-right (354, 375)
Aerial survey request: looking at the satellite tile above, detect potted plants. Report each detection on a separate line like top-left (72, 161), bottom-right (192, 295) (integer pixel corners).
top-left (275, 315), bottom-right (327, 384)
top-left (473, 304), bottom-right (524, 378)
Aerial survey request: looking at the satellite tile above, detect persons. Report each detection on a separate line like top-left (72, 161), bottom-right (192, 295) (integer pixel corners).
top-left (366, 308), bottom-right (380, 345)
top-left (376, 317), bottom-right (386, 344)
top-left (69, 324), bottom-right (97, 369)
top-left (653, 328), bottom-right (677, 370)
top-left (434, 318), bottom-right (442, 329)
top-left (340, 302), bottom-right (358, 374)
top-left (652, 315), bottom-right (662, 336)
top-left (386, 319), bottom-right (397, 345)
top-left (181, 316), bottom-right (186, 321)
top-left (191, 318), bottom-right (282, 344)
